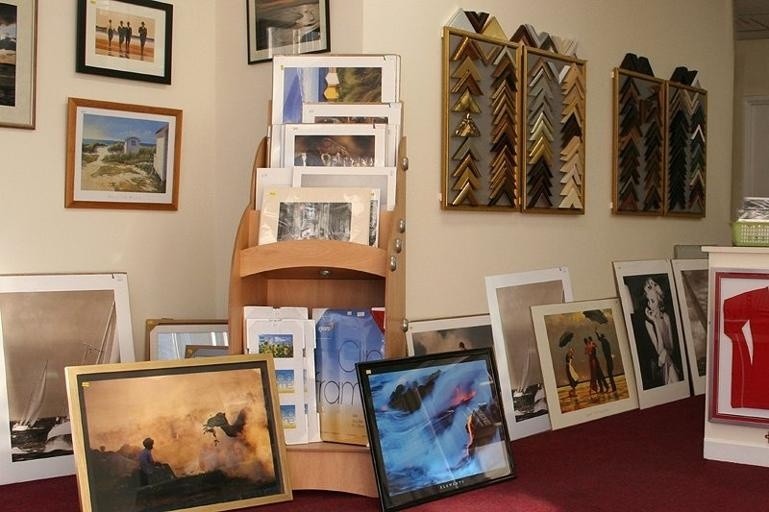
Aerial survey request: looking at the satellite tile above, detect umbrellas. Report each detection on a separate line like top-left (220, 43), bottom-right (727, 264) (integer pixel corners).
top-left (559, 330), bottom-right (576, 347)
top-left (581, 310), bottom-right (609, 329)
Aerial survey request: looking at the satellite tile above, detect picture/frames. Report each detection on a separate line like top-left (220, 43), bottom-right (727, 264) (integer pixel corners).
top-left (246, 1), bottom-right (330, 66)
top-left (0, 0), bottom-right (38, 129)
top-left (354, 346), bottom-right (521, 511)
top-left (64, 0), bottom-right (183, 211)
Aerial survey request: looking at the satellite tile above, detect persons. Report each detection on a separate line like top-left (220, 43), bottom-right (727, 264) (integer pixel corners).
top-left (644, 278), bottom-right (682, 386)
top-left (106, 18), bottom-right (114, 47)
top-left (117, 19), bottom-right (126, 50)
top-left (136, 436), bottom-right (182, 482)
top-left (583, 329), bottom-right (618, 393)
top-left (562, 345), bottom-right (581, 397)
top-left (137, 20), bottom-right (147, 56)
top-left (125, 20), bottom-right (133, 51)
top-left (457, 341), bottom-right (467, 351)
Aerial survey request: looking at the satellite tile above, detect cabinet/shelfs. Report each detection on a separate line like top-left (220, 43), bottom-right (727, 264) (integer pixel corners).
top-left (225, 135), bottom-right (409, 497)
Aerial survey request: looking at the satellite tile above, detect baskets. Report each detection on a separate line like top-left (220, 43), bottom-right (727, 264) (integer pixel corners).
top-left (729, 219), bottom-right (769, 247)
